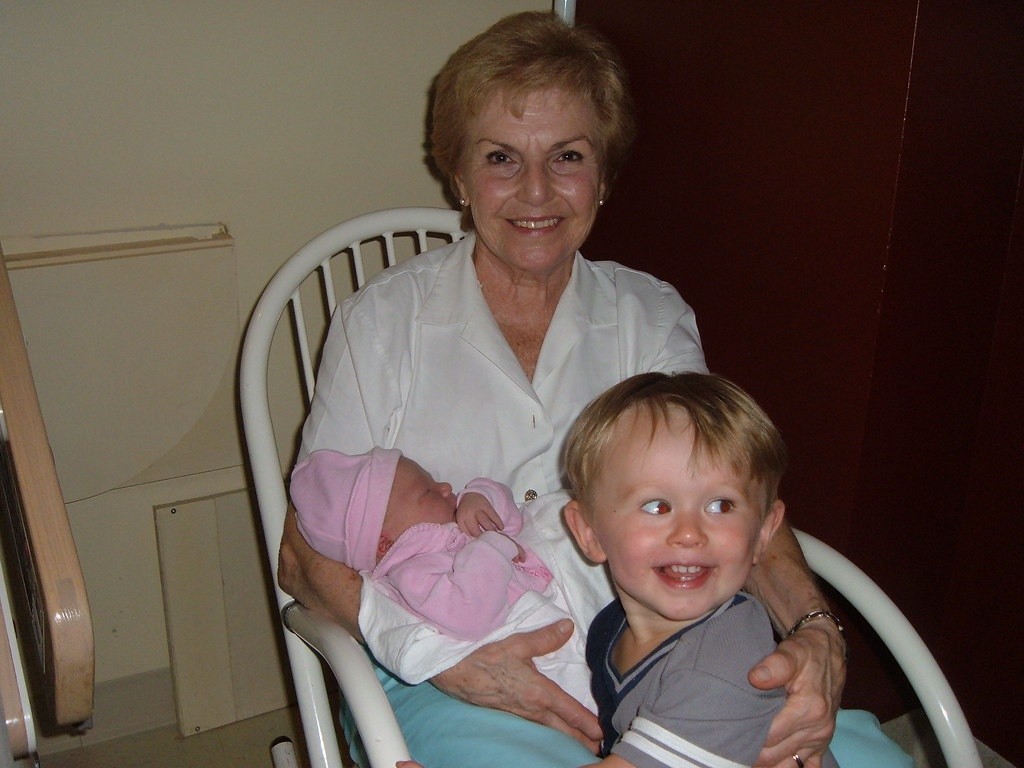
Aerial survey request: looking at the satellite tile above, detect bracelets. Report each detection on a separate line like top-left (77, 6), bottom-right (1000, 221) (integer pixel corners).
top-left (786, 609), bottom-right (847, 640)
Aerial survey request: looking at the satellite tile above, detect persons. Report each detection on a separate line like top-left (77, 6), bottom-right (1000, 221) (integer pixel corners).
top-left (561, 371), bottom-right (842, 767)
top-left (291, 446), bottom-right (599, 718)
top-left (277, 9), bottom-right (916, 768)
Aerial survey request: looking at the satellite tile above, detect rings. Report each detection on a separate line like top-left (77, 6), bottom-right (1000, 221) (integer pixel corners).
top-left (791, 753), bottom-right (805, 768)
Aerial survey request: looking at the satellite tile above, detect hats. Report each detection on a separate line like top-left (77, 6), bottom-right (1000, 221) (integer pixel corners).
top-left (290, 446), bottom-right (404, 573)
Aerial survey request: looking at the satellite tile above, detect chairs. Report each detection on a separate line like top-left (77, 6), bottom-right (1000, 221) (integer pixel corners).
top-left (240, 207), bottom-right (983, 768)
